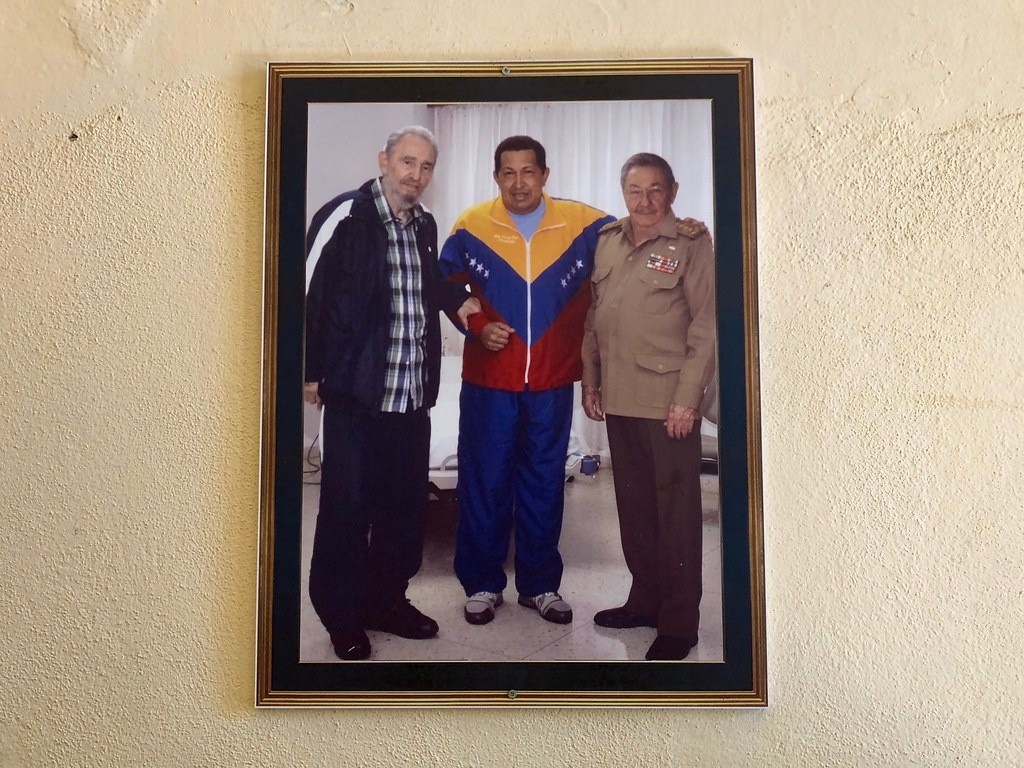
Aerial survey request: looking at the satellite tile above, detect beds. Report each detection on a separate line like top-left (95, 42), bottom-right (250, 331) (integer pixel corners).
top-left (318, 351), bottom-right (601, 490)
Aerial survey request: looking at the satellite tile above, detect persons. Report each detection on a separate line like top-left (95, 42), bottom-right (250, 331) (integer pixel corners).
top-left (305, 125), bottom-right (482, 660)
top-left (438, 135), bottom-right (712, 625)
top-left (582, 153), bottom-right (714, 661)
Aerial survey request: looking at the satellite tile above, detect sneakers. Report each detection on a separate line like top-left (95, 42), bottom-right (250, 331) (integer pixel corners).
top-left (594, 607), bottom-right (656, 629)
top-left (372, 598), bottom-right (439, 639)
top-left (644, 635), bottom-right (699, 660)
top-left (464, 590), bottom-right (503, 625)
top-left (329, 626), bottom-right (372, 661)
top-left (517, 591), bottom-right (573, 624)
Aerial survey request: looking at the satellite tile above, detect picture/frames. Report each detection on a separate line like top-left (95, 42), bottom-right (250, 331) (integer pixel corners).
top-left (256, 60), bottom-right (772, 715)
